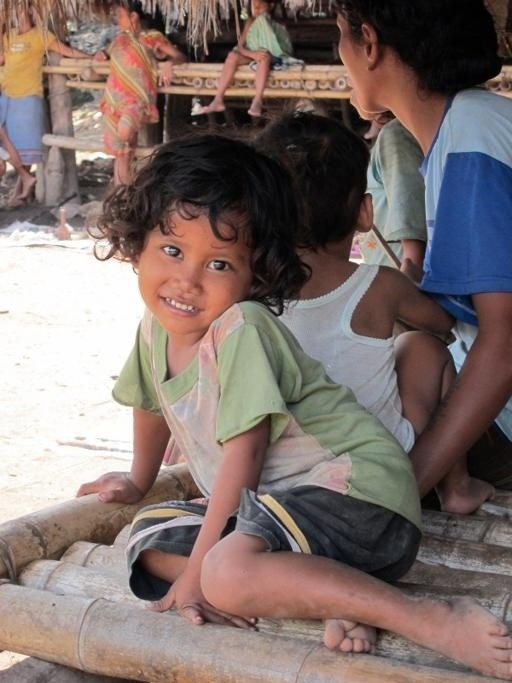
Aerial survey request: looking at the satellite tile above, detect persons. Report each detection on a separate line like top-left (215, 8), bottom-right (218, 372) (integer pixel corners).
top-left (76, 135), bottom-right (512, 683)
top-left (96, 0), bottom-right (185, 183)
top-left (200, 0), bottom-right (279, 117)
top-left (344, 77), bottom-right (426, 278)
top-left (248, 112), bottom-right (497, 514)
top-left (334, 1), bottom-right (512, 503)
top-left (0, 12), bottom-right (92, 207)
